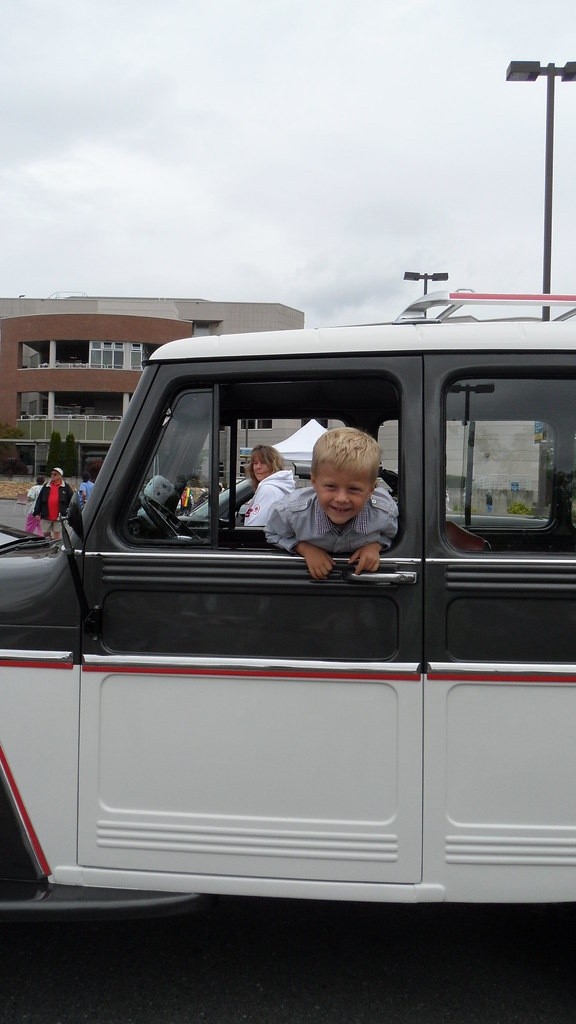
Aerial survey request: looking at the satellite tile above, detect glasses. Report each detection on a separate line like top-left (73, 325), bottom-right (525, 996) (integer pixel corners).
top-left (50, 473), bottom-right (60, 476)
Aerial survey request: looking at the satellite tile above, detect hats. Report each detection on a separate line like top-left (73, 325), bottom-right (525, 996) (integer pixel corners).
top-left (50, 468), bottom-right (63, 476)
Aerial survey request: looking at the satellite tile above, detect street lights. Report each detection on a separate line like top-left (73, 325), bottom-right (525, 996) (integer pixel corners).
top-left (507, 60), bottom-right (576, 321)
top-left (448, 383), bottom-right (495, 422)
top-left (404, 271), bottom-right (449, 318)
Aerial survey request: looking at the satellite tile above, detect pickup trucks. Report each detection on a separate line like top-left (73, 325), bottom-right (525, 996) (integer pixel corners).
top-left (176, 462), bottom-right (549, 528)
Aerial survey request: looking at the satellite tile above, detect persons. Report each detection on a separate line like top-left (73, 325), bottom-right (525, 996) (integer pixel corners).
top-left (485, 490), bottom-right (493, 513)
top-left (265, 427), bottom-right (399, 580)
top-left (25, 476), bottom-right (45, 537)
top-left (78, 472), bottom-right (95, 511)
top-left (33, 467), bottom-right (74, 539)
top-left (446, 490), bottom-right (452, 511)
top-left (181, 486), bottom-right (189, 511)
top-left (244, 444), bottom-right (297, 526)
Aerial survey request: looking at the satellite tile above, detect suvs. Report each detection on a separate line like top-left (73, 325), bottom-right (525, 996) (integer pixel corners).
top-left (0, 289), bottom-right (576, 923)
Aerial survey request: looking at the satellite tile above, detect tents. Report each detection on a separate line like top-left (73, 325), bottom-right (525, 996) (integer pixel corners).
top-left (265, 420), bottom-right (328, 467)
